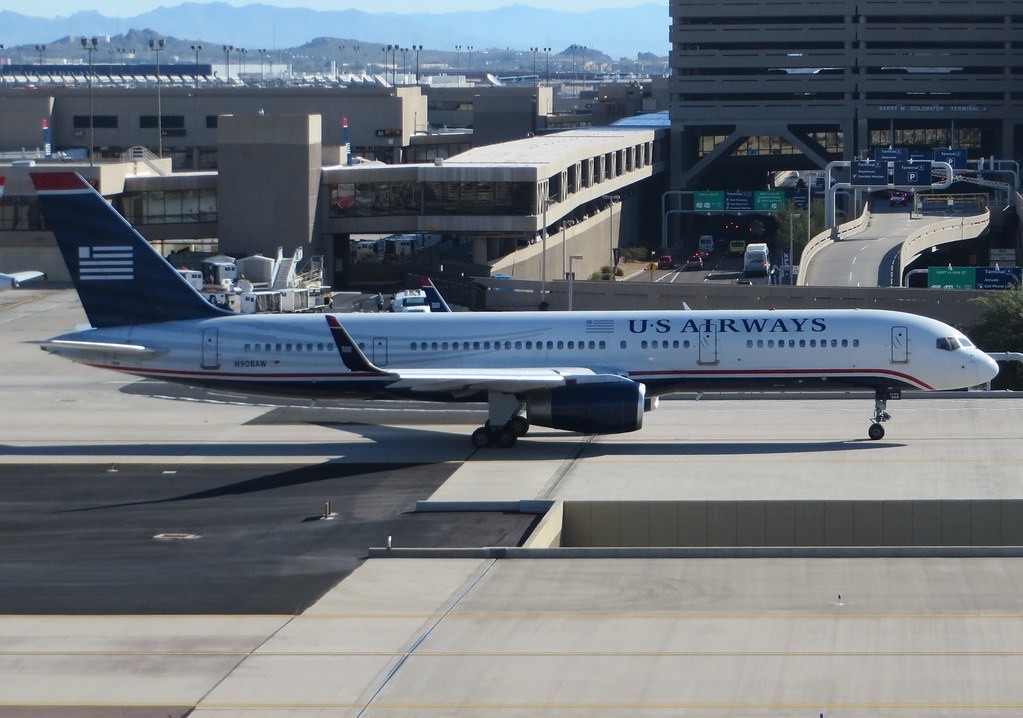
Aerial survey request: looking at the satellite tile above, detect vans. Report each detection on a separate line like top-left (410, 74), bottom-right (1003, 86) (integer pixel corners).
top-left (698, 236), bottom-right (715, 254)
top-left (730, 240), bottom-right (746, 257)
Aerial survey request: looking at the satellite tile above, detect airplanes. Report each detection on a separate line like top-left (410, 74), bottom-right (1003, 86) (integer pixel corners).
top-left (23, 170), bottom-right (1023, 455)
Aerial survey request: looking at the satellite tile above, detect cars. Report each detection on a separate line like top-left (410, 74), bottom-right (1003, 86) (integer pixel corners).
top-left (658, 256), bottom-right (671, 267)
top-left (694, 248), bottom-right (711, 261)
top-left (889, 191), bottom-right (907, 208)
top-left (686, 256), bottom-right (704, 271)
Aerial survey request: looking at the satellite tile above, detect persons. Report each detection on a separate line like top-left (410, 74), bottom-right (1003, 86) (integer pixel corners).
top-left (239, 273), bottom-right (245, 279)
top-left (328, 296), bottom-right (334, 308)
top-left (375, 292), bottom-right (384, 313)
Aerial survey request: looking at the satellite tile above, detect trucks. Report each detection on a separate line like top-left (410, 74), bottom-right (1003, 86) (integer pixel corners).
top-left (396, 289), bottom-right (431, 313)
top-left (742, 243), bottom-right (771, 277)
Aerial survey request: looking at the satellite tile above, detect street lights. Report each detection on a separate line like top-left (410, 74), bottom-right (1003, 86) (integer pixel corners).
top-left (81, 37), bottom-right (99, 169)
top-left (257, 48), bottom-right (267, 85)
top-left (806, 171), bottom-right (819, 244)
top-left (455, 44), bottom-right (461, 86)
top-left (35, 43), bottom-right (45, 80)
top-left (562, 220), bottom-right (574, 280)
top-left (571, 45), bottom-right (586, 99)
top-left (411, 44), bottom-right (425, 87)
top-left (467, 45), bottom-right (474, 87)
top-left (338, 44), bottom-right (360, 73)
top-left (569, 256), bottom-right (584, 312)
top-left (540, 200), bottom-right (555, 304)
top-left (189, 44), bottom-right (203, 88)
top-left (237, 47), bottom-right (249, 85)
top-left (529, 46), bottom-right (551, 89)
top-left (790, 212), bottom-right (802, 287)
top-left (148, 38), bottom-right (165, 156)
top-left (603, 194), bottom-right (621, 283)
top-left (222, 45), bottom-right (235, 84)
top-left (382, 44), bottom-right (408, 88)
